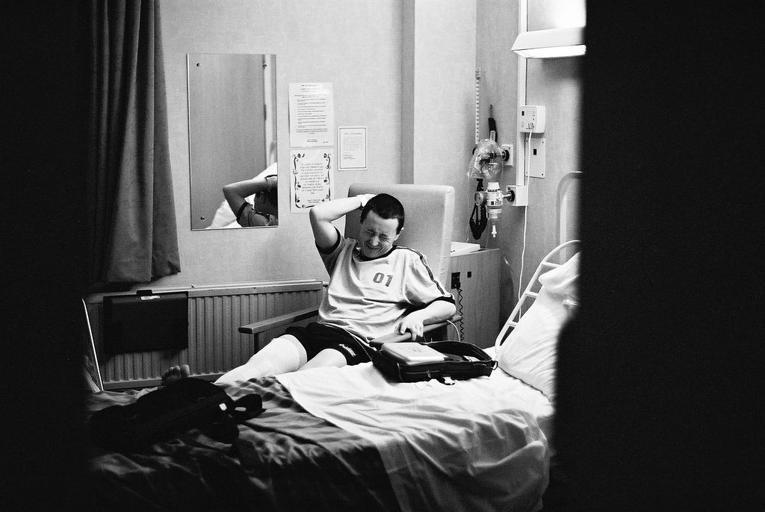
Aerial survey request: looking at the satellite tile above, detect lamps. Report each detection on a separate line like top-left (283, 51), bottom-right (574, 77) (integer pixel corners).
top-left (511, 26), bottom-right (587, 59)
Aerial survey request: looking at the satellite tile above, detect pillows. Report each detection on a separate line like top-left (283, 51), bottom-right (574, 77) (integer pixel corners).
top-left (497, 277), bottom-right (576, 404)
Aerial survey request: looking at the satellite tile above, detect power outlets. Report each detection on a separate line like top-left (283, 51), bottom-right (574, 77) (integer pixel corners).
top-left (499, 145), bottom-right (514, 168)
top-left (504, 183), bottom-right (529, 209)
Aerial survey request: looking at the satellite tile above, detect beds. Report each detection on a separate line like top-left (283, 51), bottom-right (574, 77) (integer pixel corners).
top-left (85, 170), bottom-right (582, 511)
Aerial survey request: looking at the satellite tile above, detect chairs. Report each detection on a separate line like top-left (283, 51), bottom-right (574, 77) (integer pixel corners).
top-left (238, 182), bottom-right (455, 354)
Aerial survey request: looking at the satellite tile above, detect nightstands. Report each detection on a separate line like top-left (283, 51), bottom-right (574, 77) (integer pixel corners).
top-left (447, 240), bottom-right (502, 350)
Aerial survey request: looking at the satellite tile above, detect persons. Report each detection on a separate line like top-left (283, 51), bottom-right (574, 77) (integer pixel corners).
top-left (223, 174), bottom-right (279, 228)
top-left (161, 193), bottom-right (456, 396)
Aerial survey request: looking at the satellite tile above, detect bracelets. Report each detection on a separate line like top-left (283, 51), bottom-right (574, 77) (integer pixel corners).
top-left (358, 195), bottom-right (367, 210)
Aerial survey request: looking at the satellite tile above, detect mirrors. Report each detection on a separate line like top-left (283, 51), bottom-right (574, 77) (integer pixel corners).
top-left (186, 51), bottom-right (278, 230)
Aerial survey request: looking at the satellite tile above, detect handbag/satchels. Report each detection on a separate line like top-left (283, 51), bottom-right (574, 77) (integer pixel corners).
top-left (373, 340), bottom-right (498, 383)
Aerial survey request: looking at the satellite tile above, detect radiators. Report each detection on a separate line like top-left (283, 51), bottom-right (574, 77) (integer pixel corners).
top-left (87, 281), bottom-right (324, 390)
